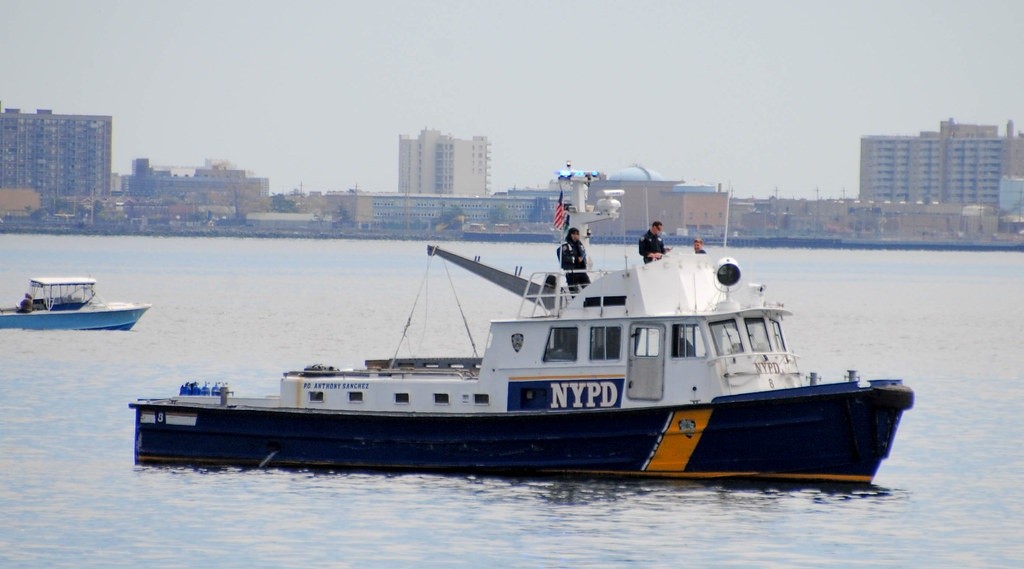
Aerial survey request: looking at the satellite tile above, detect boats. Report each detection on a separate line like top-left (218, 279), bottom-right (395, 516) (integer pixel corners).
top-left (0, 277), bottom-right (151, 327)
top-left (128, 169), bottom-right (916, 485)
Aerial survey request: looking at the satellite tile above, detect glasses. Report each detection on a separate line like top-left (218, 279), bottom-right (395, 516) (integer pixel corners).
top-left (655, 225), bottom-right (661, 232)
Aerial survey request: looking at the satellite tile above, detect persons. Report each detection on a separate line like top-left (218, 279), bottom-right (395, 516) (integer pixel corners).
top-left (639, 220), bottom-right (668, 263)
top-left (694, 239), bottom-right (705, 254)
top-left (557, 227), bottom-right (591, 300)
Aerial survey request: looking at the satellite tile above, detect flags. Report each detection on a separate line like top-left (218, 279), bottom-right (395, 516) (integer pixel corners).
top-left (553, 191), bottom-right (570, 233)
